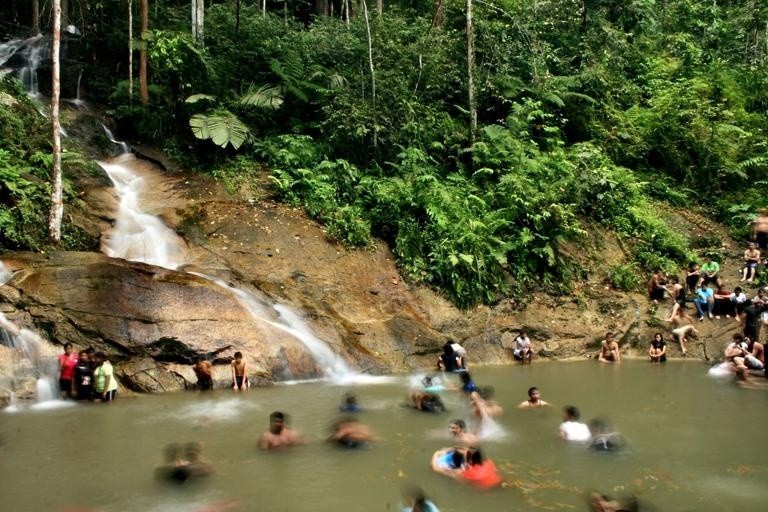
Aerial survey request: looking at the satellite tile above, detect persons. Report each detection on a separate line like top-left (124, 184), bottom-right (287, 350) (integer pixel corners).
top-left (257, 333), bottom-right (641, 512)
top-left (598, 209), bottom-right (768, 379)
top-left (194, 353), bottom-right (214, 392)
top-left (154, 438), bottom-right (216, 488)
top-left (58, 342), bottom-right (117, 405)
top-left (231, 352), bottom-right (251, 391)
